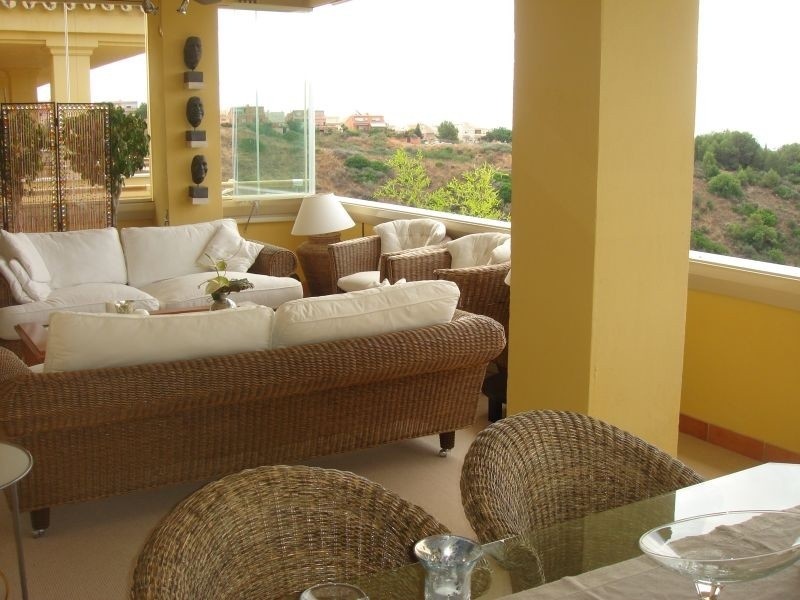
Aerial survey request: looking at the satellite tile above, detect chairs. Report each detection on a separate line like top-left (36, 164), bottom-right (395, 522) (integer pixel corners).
top-left (132, 464), bottom-right (492, 600)
top-left (326, 218), bottom-right (449, 296)
top-left (460, 407), bottom-right (704, 594)
top-left (386, 231), bottom-right (511, 375)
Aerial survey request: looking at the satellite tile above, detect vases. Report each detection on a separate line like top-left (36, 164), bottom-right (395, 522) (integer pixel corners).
top-left (209, 292), bottom-right (237, 313)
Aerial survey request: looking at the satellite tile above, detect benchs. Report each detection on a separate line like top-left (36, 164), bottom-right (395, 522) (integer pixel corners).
top-left (0, 216), bottom-right (304, 367)
top-left (0, 281), bottom-right (505, 539)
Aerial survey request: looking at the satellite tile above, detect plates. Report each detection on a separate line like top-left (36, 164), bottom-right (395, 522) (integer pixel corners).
top-left (639, 509), bottom-right (799, 585)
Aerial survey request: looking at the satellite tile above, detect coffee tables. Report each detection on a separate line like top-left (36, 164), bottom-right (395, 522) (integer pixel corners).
top-left (13, 301), bottom-right (210, 367)
top-left (0, 442), bottom-right (33, 600)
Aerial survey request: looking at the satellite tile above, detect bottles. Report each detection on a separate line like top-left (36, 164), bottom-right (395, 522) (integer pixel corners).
top-left (414, 534), bottom-right (483, 600)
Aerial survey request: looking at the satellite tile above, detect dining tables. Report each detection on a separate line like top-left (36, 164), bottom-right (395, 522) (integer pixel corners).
top-left (284, 459), bottom-right (800, 600)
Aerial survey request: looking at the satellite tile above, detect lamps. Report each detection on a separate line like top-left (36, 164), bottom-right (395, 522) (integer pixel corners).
top-left (291, 193), bottom-right (357, 297)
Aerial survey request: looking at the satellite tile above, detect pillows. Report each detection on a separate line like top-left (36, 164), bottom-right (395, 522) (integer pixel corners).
top-left (196, 224), bottom-right (264, 274)
top-left (0, 229), bottom-right (52, 283)
top-left (10, 257), bottom-right (51, 301)
